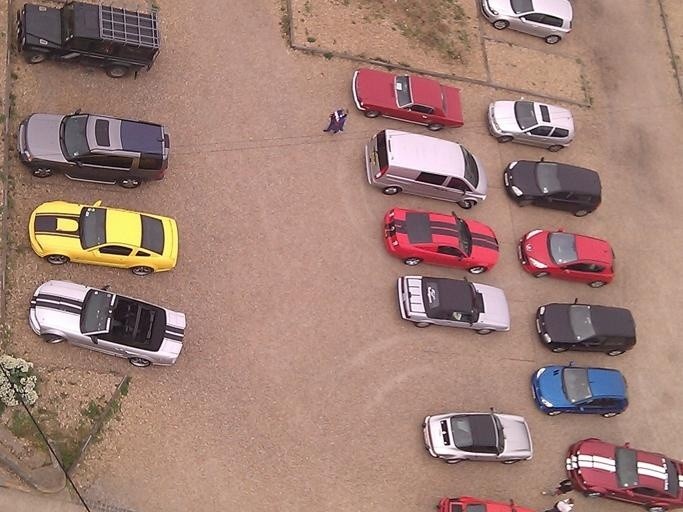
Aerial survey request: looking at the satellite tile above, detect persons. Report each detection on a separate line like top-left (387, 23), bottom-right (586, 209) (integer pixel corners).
top-left (322, 106), bottom-right (343, 133)
top-left (332, 108), bottom-right (349, 134)
top-left (543, 495), bottom-right (575, 512)
top-left (541, 478), bottom-right (578, 498)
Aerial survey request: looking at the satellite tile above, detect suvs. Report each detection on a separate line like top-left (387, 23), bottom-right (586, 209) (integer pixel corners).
top-left (16, 2), bottom-right (160, 78)
top-left (13, 108), bottom-right (170, 189)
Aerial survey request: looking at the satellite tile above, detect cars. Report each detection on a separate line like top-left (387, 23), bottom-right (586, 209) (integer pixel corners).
top-left (519, 228), bottom-right (615, 288)
top-left (29, 199), bottom-right (178, 276)
top-left (423, 407), bottom-right (532, 464)
top-left (566, 437), bottom-right (683, 512)
top-left (28, 280), bottom-right (187, 368)
top-left (530, 360), bottom-right (629, 419)
top-left (480, 0), bottom-right (573, 44)
top-left (397, 275), bottom-right (510, 335)
top-left (503, 156), bottom-right (602, 217)
top-left (385, 208), bottom-right (500, 275)
top-left (488, 97), bottom-right (575, 152)
top-left (535, 298), bottom-right (636, 356)
top-left (436, 496), bottom-right (541, 512)
top-left (352, 67), bottom-right (464, 131)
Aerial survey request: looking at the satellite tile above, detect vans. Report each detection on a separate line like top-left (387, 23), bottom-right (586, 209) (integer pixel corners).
top-left (365, 129), bottom-right (487, 209)
